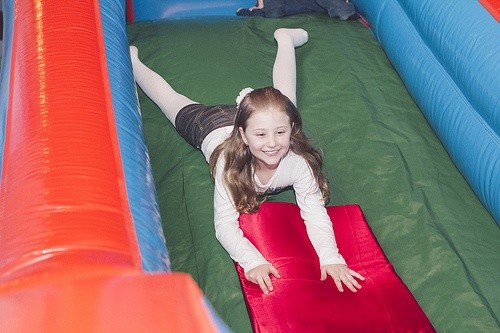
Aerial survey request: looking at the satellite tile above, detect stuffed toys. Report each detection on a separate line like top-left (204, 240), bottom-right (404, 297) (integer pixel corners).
top-left (236, 0), bottom-right (356, 21)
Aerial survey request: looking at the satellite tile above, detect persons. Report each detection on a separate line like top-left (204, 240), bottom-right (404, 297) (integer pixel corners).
top-left (129, 28), bottom-right (365, 296)
